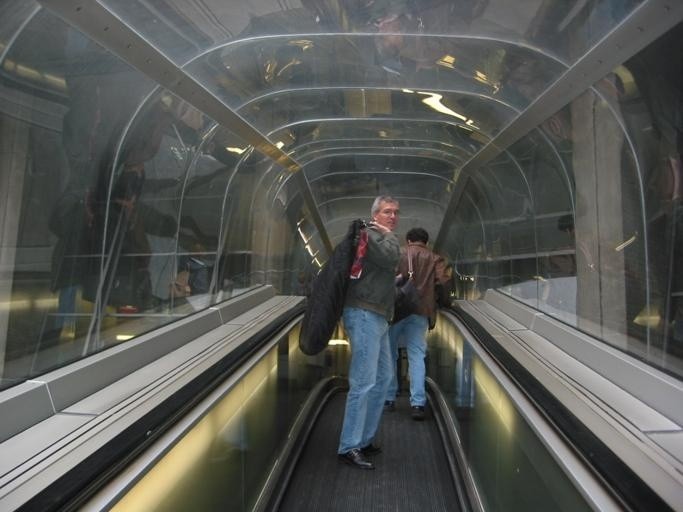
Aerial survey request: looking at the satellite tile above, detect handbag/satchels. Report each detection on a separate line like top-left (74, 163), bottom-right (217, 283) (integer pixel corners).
top-left (392, 275), bottom-right (423, 324)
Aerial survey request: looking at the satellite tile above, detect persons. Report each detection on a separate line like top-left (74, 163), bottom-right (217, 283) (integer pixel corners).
top-left (383, 225), bottom-right (455, 421)
top-left (177, 213), bottom-right (227, 296)
top-left (108, 58), bottom-right (165, 192)
top-left (544, 215), bottom-right (577, 279)
top-left (50, 145), bottom-right (106, 338)
top-left (105, 179), bottom-right (176, 309)
top-left (337, 194), bottom-right (403, 470)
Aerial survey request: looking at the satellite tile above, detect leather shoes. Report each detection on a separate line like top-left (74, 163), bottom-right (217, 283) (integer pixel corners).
top-left (384, 400), bottom-right (395, 410)
top-left (339, 442), bottom-right (382, 470)
top-left (411, 405), bottom-right (426, 420)
